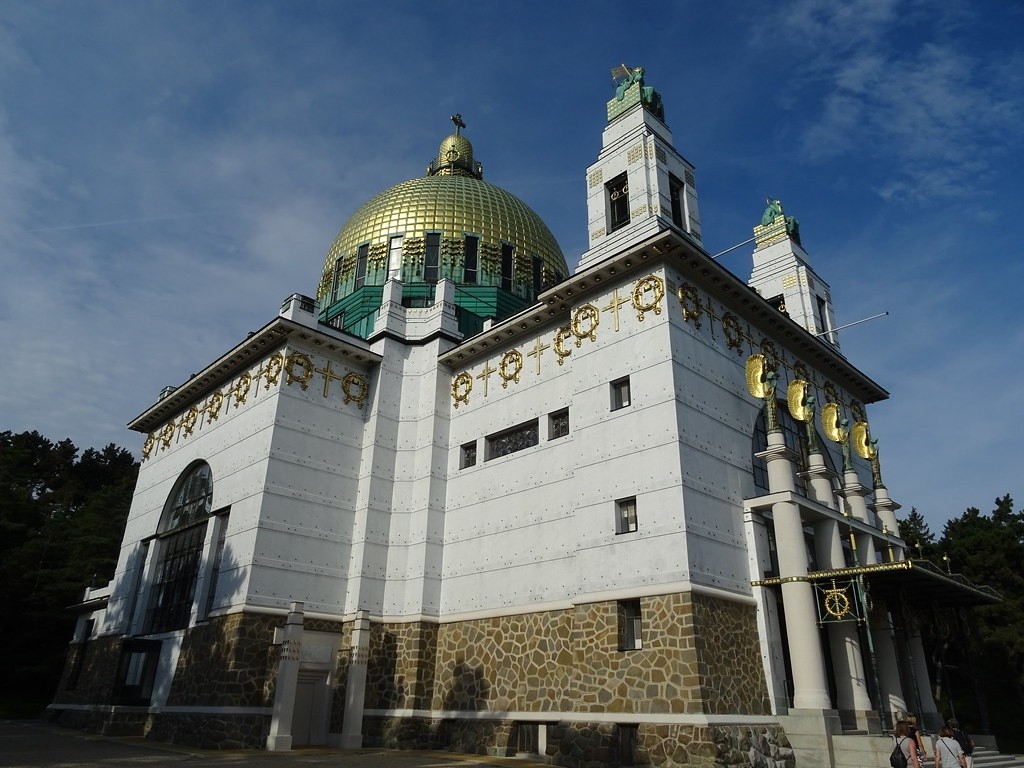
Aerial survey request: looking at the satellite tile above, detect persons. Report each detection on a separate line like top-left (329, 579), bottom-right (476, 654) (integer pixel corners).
top-left (893, 711), bottom-right (927, 768)
top-left (787, 380), bottom-right (821, 454)
top-left (745, 354), bottom-right (783, 433)
top-left (935, 719), bottom-right (975, 768)
top-left (822, 403), bottom-right (857, 474)
top-left (852, 422), bottom-right (887, 489)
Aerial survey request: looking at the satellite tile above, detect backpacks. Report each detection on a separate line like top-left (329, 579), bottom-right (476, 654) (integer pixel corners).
top-left (890, 736), bottom-right (911, 768)
top-left (954, 728), bottom-right (972, 754)
